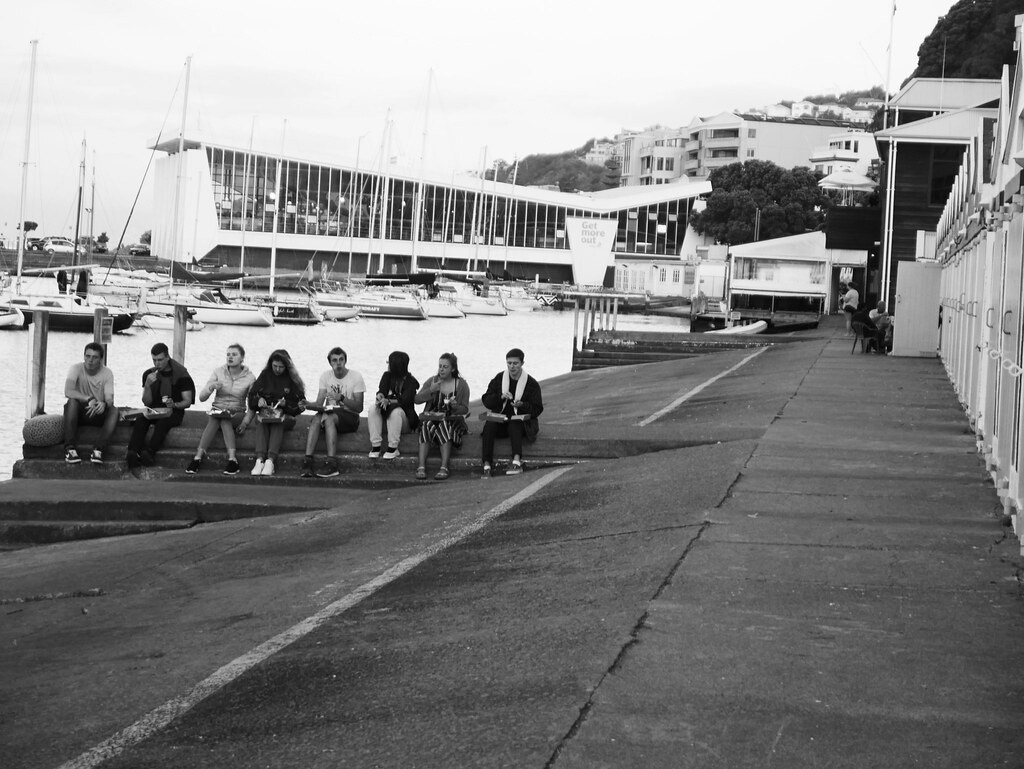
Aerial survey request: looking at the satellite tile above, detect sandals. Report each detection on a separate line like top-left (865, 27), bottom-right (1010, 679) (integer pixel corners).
top-left (434, 466), bottom-right (449, 479)
top-left (416, 466), bottom-right (426, 478)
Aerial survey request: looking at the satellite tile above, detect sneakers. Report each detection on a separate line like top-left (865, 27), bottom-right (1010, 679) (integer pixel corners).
top-left (261, 459), bottom-right (276, 475)
top-left (315, 461), bottom-right (339, 477)
top-left (506, 464), bottom-right (523, 474)
top-left (65, 448), bottom-right (81, 462)
top-left (251, 459), bottom-right (265, 476)
top-left (186, 457), bottom-right (200, 473)
top-left (223, 460), bottom-right (240, 474)
top-left (300, 463), bottom-right (314, 476)
top-left (481, 468), bottom-right (490, 479)
top-left (91, 448), bottom-right (102, 463)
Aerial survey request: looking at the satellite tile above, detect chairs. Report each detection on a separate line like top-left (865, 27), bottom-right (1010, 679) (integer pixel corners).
top-left (851, 322), bottom-right (886, 355)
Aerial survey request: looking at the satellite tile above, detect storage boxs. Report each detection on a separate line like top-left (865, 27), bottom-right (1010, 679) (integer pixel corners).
top-left (121, 407), bottom-right (173, 419)
top-left (479, 411), bottom-right (507, 422)
top-left (303, 403), bottom-right (344, 412)
top-left (257, 408), bottom-right (286, 423)
top-left (511, 414), bottom-right (531, 420)
top-left (419, 411), bottom-right (471, 421)
top-left (205, 408), bottom-right (236, 418)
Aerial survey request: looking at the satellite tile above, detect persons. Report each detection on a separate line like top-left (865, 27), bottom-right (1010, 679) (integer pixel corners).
top-left (482, 349), bottom-right (543, 478)
top-left (869, 301), bottom-right (890, 330)
top-left (299, 348), bottom-right (366, 477)
top-left (63, 342), bottom-right (119, 463)
top-left (843, 282), bottom-right (858, 335)
top-left (415, 353), bottom-right (470, 479)
top-left (127, 343), bottom-right (196, 467)
top-left (368, 350), bottom-right (419, 459)
top-left (186, 344), bottom-right (255, 474)
top-left (248, 350), bottom-right (306, 475)
top-left (57, 265), bottom-right (67, 293)
top-left (851, 303), bottom-right (885, 351)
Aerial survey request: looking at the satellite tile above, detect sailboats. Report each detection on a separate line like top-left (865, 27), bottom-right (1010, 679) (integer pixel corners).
top-left (0, 38), bottom-right (552, 333)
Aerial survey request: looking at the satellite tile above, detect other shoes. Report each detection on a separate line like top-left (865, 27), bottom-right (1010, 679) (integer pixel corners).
top-left (141, 449), bottom-right (156, 465)
top-left (369, 447), bottom-right (381, 458)
top-left (128, 450), bottom-right (140, 468)
top-left (383, 448), bottom-right (400, 459)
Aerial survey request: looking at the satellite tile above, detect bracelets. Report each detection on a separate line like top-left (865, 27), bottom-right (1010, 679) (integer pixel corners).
top-left (88, 397), bottom-right (96, 403)
top-left (103, 401), bottom-right (108, 408)
top-left (388, 399), bottom-right (391, 405)
top-left (175, 402), bottom-right (177, 408)
top-left (339, 394), bottom-right (345, 401)
